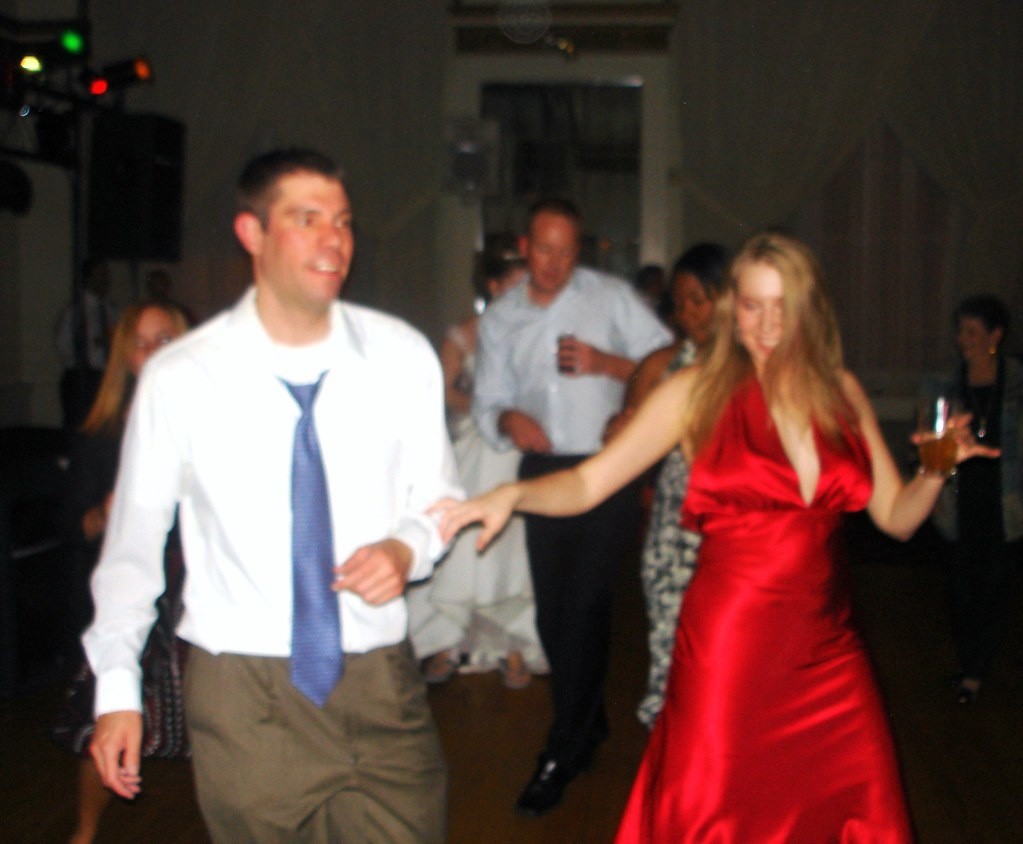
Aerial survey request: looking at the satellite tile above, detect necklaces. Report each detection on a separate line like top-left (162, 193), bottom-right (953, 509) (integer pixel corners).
top-left (968, 388), bottom-right (992, 438)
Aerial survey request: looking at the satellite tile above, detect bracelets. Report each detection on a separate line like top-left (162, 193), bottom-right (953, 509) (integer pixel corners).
top-left (918, 464), bottom-right (958, 478)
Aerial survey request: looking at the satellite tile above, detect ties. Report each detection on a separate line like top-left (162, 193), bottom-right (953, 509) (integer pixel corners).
top-left (278, 370), bottom-right (344, 708)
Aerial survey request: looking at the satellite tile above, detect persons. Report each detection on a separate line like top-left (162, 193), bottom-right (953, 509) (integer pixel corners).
top-left (434, 199), bottom-right (685, 821)
top-left (911, 292), bottom-right (1023, 710)
top-left (56, 261), bottom-right (116, 429)
top-left (422, 231), bottom-right (1001, 844)
top-left (76, 145), bottom-right (468, 844)
top-left (603, 240), bottom-right (738, 741)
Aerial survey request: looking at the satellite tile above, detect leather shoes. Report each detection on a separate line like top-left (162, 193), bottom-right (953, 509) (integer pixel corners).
top-left (514, 723), bottom-right (610, 819)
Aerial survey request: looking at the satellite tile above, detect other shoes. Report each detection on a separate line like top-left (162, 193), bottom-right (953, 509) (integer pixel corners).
top-left (424, 657), bottom-right (459, 683)
top-left (502, 662), bottom-right (533, 687)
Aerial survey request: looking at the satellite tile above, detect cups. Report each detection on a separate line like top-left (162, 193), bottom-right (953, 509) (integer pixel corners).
top-left (555, 326), bottom-right (578, 381)
top-left (916, 398), bottom-right (959, 480)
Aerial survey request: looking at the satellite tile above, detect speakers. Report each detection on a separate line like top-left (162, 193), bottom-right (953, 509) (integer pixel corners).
top-left (85, 112), bottom-right (186, 265)
